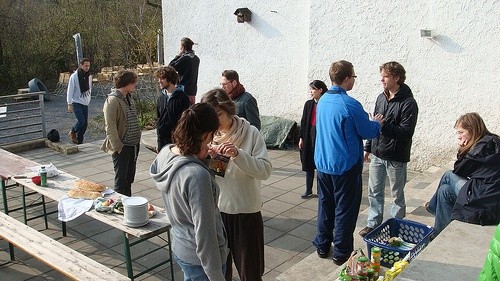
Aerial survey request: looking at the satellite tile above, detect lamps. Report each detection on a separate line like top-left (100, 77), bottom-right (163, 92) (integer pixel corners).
top-left (420, 28), bottom-right (438, 39)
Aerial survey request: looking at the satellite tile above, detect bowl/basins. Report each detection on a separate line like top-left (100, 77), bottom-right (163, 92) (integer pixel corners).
top-left (31, 175), bottom-right (41, 185)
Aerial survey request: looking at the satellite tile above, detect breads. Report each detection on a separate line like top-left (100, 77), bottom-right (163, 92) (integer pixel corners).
top-left (76, 180), bottom-right (106, 191)
top-left (67, 189), bottom-right (102, 199)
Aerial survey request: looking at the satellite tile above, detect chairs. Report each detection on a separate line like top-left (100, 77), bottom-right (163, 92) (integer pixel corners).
top-left (54, 62), bottom-right (164, 101)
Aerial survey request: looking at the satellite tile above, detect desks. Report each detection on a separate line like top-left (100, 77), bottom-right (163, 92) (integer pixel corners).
top-left (0, 148), bottom-right (172, 281)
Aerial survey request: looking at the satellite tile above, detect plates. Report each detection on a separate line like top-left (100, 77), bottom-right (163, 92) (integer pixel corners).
top-left (122, 196), bottom-right (150, 227)
top-left (68, 190), bottom-right (103, 200)
top-left (76, 184), bottom-right (109, 192)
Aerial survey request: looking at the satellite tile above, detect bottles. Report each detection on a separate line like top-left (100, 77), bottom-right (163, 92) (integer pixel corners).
top-left (39, 165), bottom-right (48, 186)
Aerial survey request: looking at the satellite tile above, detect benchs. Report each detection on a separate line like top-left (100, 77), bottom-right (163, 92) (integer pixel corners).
top-left (393, 220), bottom-right (497, 281)
top-left (0, 210), bottom-right (133, 281)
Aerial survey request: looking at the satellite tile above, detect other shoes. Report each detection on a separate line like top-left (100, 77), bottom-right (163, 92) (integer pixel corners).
top-left (358, 227), bottom-right (374, 238)
top-left (301, 190), bottom-right (313, 199)
top-left (69, 130), bottom-right (79, 144)
top-left (316, 247), bottom-right (330, 258)
top-left (426, 202), bottom-right (436, 216)
top-left (332, 255), bottom-right (349, 266)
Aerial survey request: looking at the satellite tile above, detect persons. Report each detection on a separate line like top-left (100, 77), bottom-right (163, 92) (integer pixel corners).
top-left (297, 80), bottom-right (328, 199)
top-left (359, 61), bottom-right (418, 237)
top-left (103, 70), bottom-right (142, 197)
top-left (168, 38), bottom-right (200, 105)
top-left (220, 70), bottom-right (261, 131)
top-left (312, 60), bottom-right (384, 266)
top-left (152, 68), bottom-right (190, 154)
top-left (150, 103), bottom-right (230, 281)
top-left (200, 89), bottom-right (272, 281)
top-left (425, 112), bottom-right (500, 242)
top-left (66, 58), bottom-right (92, 145)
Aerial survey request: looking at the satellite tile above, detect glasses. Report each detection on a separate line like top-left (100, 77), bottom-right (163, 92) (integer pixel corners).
top-left (220, 81), bottom-right (232, 86)
top-left (345, 75), bottom-right (358, 79)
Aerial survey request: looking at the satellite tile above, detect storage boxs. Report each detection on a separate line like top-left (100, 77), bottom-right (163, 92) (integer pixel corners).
top-left (363, 218), bottom-right (434, 268)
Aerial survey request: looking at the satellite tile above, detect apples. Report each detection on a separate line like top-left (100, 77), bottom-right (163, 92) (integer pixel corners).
top-left (101, 199), bottom-right (114, 206)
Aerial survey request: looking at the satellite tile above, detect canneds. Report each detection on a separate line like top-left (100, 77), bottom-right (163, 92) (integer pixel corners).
top-left (356, 247), bottom-right (381, 281)
